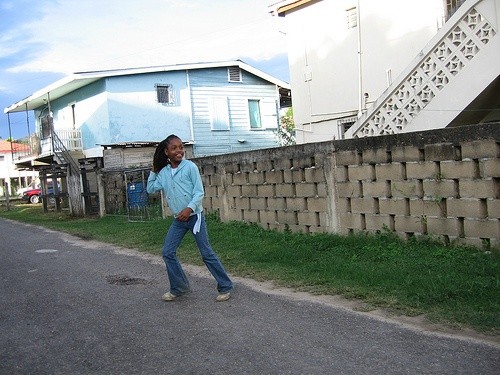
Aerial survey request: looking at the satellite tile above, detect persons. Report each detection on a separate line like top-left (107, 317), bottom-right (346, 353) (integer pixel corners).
top-left (146, 134), bottom-right (235, 302)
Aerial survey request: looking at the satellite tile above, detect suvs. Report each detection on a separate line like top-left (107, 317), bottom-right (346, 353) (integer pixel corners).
top-left (23, 180), bottom-right (60, 205)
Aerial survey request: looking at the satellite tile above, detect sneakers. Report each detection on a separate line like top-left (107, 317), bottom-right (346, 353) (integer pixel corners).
top-left (214, 293), bottom-right (230, 301)
top-left (162, 291), bottom-right (177, 301)
